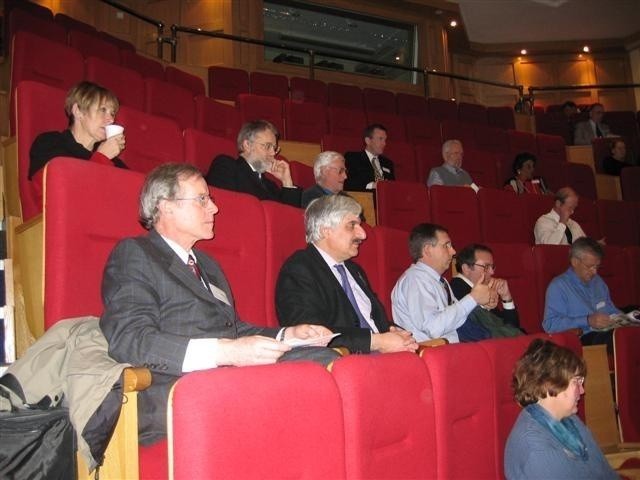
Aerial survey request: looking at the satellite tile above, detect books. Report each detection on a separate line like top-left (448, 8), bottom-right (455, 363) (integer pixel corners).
top-left (590, 310), bottom-right (640, 332)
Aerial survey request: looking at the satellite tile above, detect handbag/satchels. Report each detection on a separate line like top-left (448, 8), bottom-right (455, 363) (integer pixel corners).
top-left (1, 408), bottom-right (77, 479)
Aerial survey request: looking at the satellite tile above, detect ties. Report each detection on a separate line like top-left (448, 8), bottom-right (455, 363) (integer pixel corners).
top-left (188, 255), bottom-right (201, 281)
top-left (596, 125), bottom-right (603, 138)
top-left (334, 265), bottom-right (375, 334)
top-left (441, 278), bottom-right (451, 305)
top-left (371, 158), bottom-right (381, 181)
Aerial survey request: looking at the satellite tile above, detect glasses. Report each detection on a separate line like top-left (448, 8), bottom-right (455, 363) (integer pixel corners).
top-left (467, 263), bottom-right (496, 272)
top-left (569, 377), bottom-right (585, 385)
top-left (328, 167), bottom-right (348, 175)
top-left (578, 257), bottom-right (603, 269)
top-left (167, 194), bottom-right (209, 207)
top-left (252, 142), bottom-right (281, 154)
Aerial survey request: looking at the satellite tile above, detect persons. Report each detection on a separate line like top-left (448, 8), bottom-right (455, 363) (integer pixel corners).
top-left (205, 118), bottom-right (302, 208)
top-left (342, 124), bottom-right (396, 206)
top-left (389, 223), bottom-right (500, 344)
top-left (300, 151), bottom-right (366, 221)
top-left (29, 80), bottom-right (128, 182)
top-left (542, 236), bottom-right (640, 354)
top-left (426, 138), bottom-right (484, 194)
top-left (449, 243), bottom-right (529, 342)
top-left (274, 195), bottom-right (419, 355)
top-left (532, 187), bottom-right (607, 247)
top-left (99, 163), bottom-right (342, 448)
top-left (510, 92), bottom-right (638, 177)
top-left (503, 154), bottom-right (554, 195)
top-left (502, 339), bottom-right (622, 480)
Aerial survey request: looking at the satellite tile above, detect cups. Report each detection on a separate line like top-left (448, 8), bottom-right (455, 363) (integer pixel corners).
top-left (105, 125), bottom-right (124, 140)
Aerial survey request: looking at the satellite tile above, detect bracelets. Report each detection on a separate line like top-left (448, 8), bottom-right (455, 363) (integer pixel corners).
top-left (279, 326), bottom-right (289, 343)
top-left (501, 297), bottom-right (514, 304)
